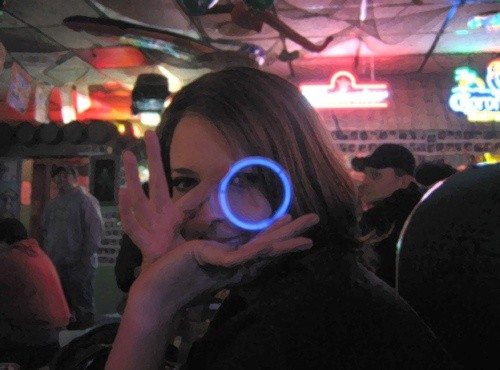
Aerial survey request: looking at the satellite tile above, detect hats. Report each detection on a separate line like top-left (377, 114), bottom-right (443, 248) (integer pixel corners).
top-left (351, 144), bottom-right (416, 175)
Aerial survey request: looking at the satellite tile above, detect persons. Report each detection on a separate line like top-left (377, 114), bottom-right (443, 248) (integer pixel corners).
top-left (351, 144), bottom-right (426, 287)
top-left (54, 66), bottom-right (454, 370)
top-left (41, 167), bottom-right (104, 330)
top-left (0, 217), bottom-right (72, 332)
